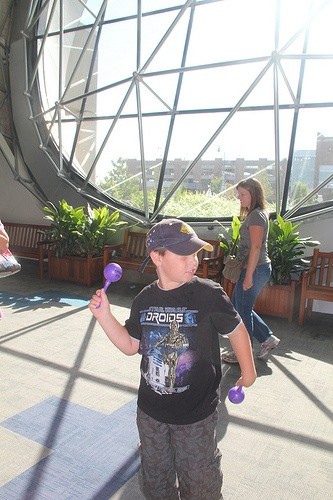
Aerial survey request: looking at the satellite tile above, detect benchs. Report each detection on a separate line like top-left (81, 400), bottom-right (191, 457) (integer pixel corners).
top-left (2, 222), bottom-right (58, 280)
top-left (103, 230), bottom-right (226, 287)
top-left (296, 248), bottom-right (333, 326)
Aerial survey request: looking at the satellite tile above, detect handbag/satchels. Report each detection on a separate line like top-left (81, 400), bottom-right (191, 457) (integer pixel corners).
top-left (0, 249), bottom-right (21, 279)
top-left (222, 256), bottom-right (242, 282)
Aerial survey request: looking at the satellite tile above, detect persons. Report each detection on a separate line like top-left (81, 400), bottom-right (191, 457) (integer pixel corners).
top-left (89, 219), bottom-right (257, 500)
top-left (221, 178), bottom-right (280, 363)
top-left (0, 220), bottom-right (9, 253)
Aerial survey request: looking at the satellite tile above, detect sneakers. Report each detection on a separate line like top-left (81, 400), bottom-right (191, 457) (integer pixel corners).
top-left (222, 351), bottom-right (239, 363)
top-left (256, 335), bottom-right (280, 357)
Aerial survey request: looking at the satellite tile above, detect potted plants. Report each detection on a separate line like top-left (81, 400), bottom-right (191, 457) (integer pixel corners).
top-left (214, 213), bottom-right (320, 324)
top-left (36, 197), bottom-right (128, 287)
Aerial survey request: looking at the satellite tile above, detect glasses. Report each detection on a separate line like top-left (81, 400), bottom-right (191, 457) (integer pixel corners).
top-left (241, 181), bottom-right (254, 187)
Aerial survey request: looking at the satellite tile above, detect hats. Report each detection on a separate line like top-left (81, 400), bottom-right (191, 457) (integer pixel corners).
top-left (146, 218), bottom-right (214, 258)
